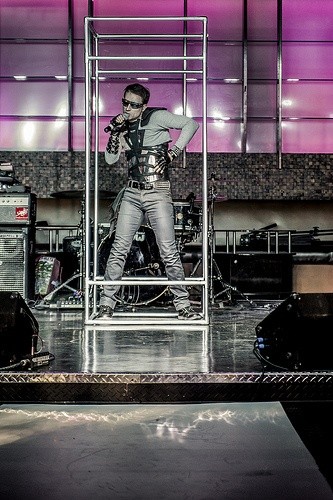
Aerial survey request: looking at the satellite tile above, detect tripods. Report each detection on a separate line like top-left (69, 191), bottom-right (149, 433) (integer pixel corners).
top-left (184, 184), bottom-right (232, 304)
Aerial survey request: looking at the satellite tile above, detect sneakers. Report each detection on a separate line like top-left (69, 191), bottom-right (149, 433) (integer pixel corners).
top-left (94, 305), bottom-right (113, 321)
top-left (178, 307), bottom-right (201, 319)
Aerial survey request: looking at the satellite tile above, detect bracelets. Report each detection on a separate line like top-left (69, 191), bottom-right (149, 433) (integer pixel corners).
top-left (168, 145), bottom-right (182, 160)
top-left (106, 130), bottom-right (119, 155)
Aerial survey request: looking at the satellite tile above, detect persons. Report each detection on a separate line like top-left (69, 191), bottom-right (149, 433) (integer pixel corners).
top-left (99, 83), bottom-right (203, 320)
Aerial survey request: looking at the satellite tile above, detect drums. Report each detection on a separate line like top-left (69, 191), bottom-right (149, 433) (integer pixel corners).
top-left (97, 224), bottom-right (169, 305)
top-left (88, 222), bottom-right (111, 254)
top-left (172, 202), bottom-right (202, 236)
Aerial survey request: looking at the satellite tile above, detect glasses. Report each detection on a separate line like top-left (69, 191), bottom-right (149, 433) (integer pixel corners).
top-left (121, 98), bottom-right (144, 110)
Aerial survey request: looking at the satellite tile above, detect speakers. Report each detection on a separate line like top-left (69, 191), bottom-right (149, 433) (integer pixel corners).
top-left (0, 226), bottom-right (36, 304)
top-left (0, 291), bottom-right (39, 367)
top-left (255, 293), bottom-right (333, 371)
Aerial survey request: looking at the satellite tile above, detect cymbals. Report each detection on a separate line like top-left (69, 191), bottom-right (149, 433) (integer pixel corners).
top-left (50, 189), bottom-right (118, 200)
top-left (194, 194), bottom-right (230, 202)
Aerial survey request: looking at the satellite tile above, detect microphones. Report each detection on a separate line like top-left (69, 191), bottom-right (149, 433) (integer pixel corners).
top-left (104, 112), bottom-right (128, 133)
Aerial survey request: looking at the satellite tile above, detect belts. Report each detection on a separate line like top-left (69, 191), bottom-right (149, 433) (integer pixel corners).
top-left (127, 181), bottom-right (154, 191)
